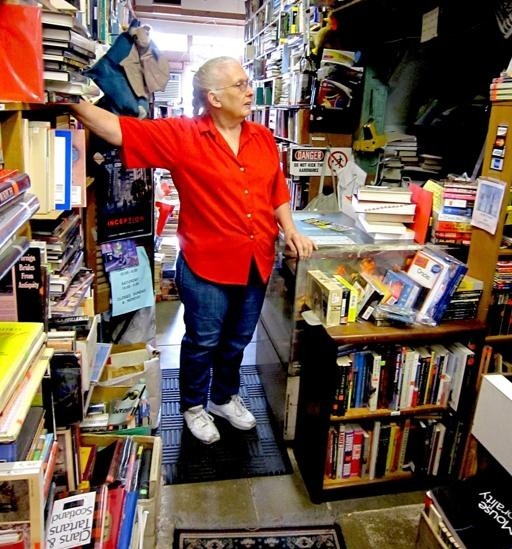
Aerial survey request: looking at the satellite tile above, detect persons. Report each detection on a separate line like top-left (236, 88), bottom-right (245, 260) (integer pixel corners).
top-left (68, 55), bottom-right (319, 445)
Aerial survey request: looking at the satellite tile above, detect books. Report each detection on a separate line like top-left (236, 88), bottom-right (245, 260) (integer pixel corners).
top-left (1, 250), bottom-right (166, 549)
top-left (2, 2), bottom-right (163, 283)
top-left (421, 375), bottom-right (512, 548)
top-left (307, 248), bottom-right (484, 329)
top-left (241, 0), bottom-right (332, 219)
top-left (343, 135), bottom-right (479, 248)
top-left (490, 204), bottom-right (512, 333)
top-left (329, 343), bottom-right (512, 483)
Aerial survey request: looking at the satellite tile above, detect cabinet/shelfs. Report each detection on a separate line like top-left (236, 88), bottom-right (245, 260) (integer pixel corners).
top-left (242, 4), bottom-right (511, 549)
top-left (0, 0), bottom-right (192, 549)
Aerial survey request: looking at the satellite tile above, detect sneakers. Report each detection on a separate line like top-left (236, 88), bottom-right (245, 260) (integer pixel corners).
top-left (207, 394), bottom-right (258, 431)
top-left (183, 404), bottom-right (221, 446)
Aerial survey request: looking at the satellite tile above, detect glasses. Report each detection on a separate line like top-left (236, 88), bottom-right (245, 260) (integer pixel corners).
top-left (215, 79), bottom-right (254, 93)
